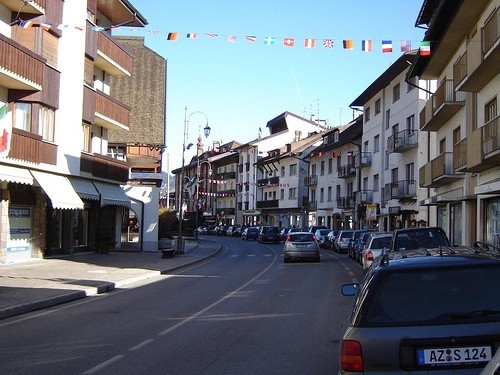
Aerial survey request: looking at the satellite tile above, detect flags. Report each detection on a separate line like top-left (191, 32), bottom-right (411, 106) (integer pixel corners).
top-left (127, 27), bottom-right (140, 36)
top-left (166, 32), bottom-right (180, 42)
top-left (184, 32), bottom-right (199, 40)
top-left (37, 21), bottom-right (53, 31)
top-left (400, 40), bottom-right (412, 52)
top-left (321, 37), bottom-right (334, 50)
top-left (341, 39), bottom-right (356, 51)
top-left (245, 34), bottom-right (257, 45)
top-left (281, 37), bottom-right (296, 47)
top-left (223, 32), bottom-right (238, 44)
top-left (361, 40), bottom-right (372, 53)
top-left (108, 26), bottom-right (122, 34)
top-left (381, 40), bottom-right (394, 53)
top-left (263, 36), bottom-right (276, 46)
top-left (15, 20), bottom-right (34, 30)
top-left (144, 28), bottom-right (159, 37)
top-left (303, 37), bottom-right (316, 49)
top-left (73, 22), bottom-right (88, 31)
top-left (90, 24), bottom-right (106, 33)
top-left (204, 31), bottom-right (217, 40)
top-left (418, 39), bottom-right (432, 57)
top-left (55, 20), bottom-right (72, 32)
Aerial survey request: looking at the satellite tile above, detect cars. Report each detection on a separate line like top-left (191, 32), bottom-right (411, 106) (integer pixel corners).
top-left (314, 230), bottom-right (379, 262)
top-left (362, 233), bottom-right (411, 274)
top-left (284, 232), bottom-right (321, 262)
top-left (196, 224), bottom-right (246, 236)
top-left (259, 225), bottom-right (279, 245)
top-left (277, 226), bottom-right (328, 249)
top-left (241, 226), bottom-right (259, 238)
top-left (389, 227), bottom-right (450, 247)
top-left (339, 246), bottom-right (500, 375)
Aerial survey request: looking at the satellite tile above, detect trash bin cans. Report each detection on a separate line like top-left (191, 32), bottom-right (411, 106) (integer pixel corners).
top-left (172, 236), bottom-right (185, 255)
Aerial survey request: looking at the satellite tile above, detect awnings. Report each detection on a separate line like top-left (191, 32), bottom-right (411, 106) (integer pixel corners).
top-left (30, 168), bottom-right (85, 210)
top-left (0, 164), bottom-right (39, 188)
top-left (68, 176), bottom-right (132, 207)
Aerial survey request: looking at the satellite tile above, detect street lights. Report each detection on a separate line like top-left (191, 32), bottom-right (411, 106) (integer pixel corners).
top-left (178, 105), bottom-right (211, 265)
